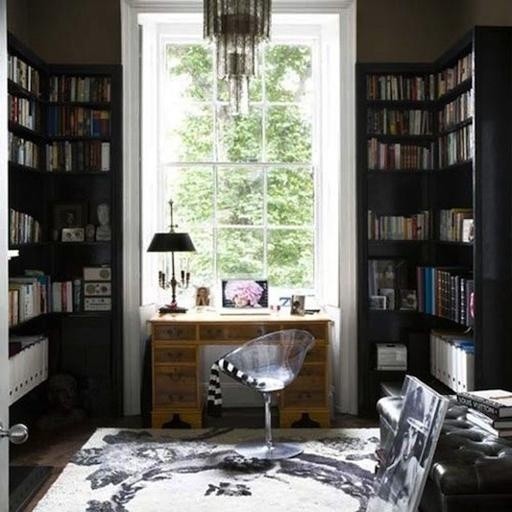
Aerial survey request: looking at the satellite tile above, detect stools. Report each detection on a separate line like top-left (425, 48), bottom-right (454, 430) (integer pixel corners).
top-left (375, 385), bottom-right (512, 512)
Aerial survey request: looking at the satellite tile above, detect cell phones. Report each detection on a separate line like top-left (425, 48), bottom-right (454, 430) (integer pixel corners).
top-left (305, 312), bottom-right (314, 315)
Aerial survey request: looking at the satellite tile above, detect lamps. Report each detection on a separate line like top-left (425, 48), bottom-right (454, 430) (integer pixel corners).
top-left (201, 0), bottom-right (273, 113)
top-left (145, 197), bottom-right (197, 313)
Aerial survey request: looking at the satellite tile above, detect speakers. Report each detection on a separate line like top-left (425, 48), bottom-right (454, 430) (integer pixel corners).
top-left (291, 295), bottom-right (305, 315)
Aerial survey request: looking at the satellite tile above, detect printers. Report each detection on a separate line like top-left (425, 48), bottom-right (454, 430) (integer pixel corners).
top-left (376, 344), bottom-right (407, 371)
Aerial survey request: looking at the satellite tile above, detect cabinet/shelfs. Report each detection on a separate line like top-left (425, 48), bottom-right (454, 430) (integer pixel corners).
top-left (353, 60), bottom-right (434, 417)
top-left (44, 61), bottom-right (124, 420)
top-left (4, 35), bottom-right (51, 409)
top-left (433, 25), bottom-right (512, 391)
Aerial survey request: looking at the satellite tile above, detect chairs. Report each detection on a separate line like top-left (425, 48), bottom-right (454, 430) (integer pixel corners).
top-left (218, 329), bottom-right (317, 462)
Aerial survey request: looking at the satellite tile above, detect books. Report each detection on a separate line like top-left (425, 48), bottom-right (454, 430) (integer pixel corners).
top-left (8, 50), bottom-right (118, 405)
top-left (366, 258), bottom-right (475, 327)
top-left (455, 388), bottom-right (512, 439)
top-left (368, 206), bottom-right (474, 243)
top-left (376, 329), bottom-right (477, 392)
top-left (364, 374), bottom-right (450, 512)
top-left (359, 53), bottom-right (476, 171)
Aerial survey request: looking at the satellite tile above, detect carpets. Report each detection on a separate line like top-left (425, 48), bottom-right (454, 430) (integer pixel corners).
top-left (29, 425), bottom-right (421, 512)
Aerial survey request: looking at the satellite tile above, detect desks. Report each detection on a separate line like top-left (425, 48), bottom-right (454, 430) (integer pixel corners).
top-left (145, 304), bottom-right (334, 427)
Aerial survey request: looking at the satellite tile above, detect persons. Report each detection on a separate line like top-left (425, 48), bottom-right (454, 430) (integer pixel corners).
top-left (223, 280), bottom-right (264, 309)
top-left (378, 387), bottom-right (433, 512)
top-left (196, 285), bottom-right (211, 308)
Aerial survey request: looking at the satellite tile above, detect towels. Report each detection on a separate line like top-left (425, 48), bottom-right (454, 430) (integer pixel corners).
top-left (206, 357), bottom-right (265, 420)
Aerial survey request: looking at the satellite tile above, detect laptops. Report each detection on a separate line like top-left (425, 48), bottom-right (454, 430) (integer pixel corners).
top-left (219, 278), bottom-right (271, 315)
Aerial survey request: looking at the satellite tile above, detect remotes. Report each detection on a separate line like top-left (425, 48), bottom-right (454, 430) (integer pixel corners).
top-left (305, 309), bottom-right (321, 312)
